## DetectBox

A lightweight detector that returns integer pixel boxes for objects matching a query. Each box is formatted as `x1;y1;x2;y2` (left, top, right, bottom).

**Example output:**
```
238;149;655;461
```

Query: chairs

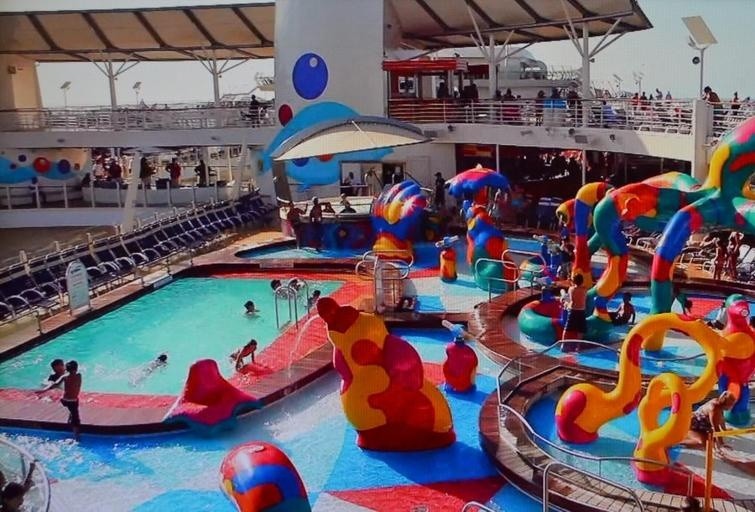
0;187;277;327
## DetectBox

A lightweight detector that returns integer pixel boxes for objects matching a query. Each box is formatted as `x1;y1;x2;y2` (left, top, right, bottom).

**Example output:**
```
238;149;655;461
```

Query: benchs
613;214;753;285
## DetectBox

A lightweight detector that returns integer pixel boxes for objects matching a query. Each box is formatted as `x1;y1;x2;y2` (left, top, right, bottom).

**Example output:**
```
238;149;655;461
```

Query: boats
375;42;587;98
131;74;279;189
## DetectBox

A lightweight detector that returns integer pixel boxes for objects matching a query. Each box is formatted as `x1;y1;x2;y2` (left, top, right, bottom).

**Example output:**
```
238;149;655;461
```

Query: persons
127;350;169;389
496;88;582;127
670;230;742;331
228;338;258;372
435;173;447;209
243;279;321;314
690;391;735;455
34;359;82;442
287;172;357;252
40;359;66;388
249;93;260;127
704;87;740;137
602;88;672;123
1;459;39;512
487;153;604;226
439;82;479;121
29;176;48;207
557;224;663;352
93;154;211;189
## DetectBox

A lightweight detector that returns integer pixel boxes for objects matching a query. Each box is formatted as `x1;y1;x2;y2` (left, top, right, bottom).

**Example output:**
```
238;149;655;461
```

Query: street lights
59;79;73;110
132;80;142;106
683;15;719;102
